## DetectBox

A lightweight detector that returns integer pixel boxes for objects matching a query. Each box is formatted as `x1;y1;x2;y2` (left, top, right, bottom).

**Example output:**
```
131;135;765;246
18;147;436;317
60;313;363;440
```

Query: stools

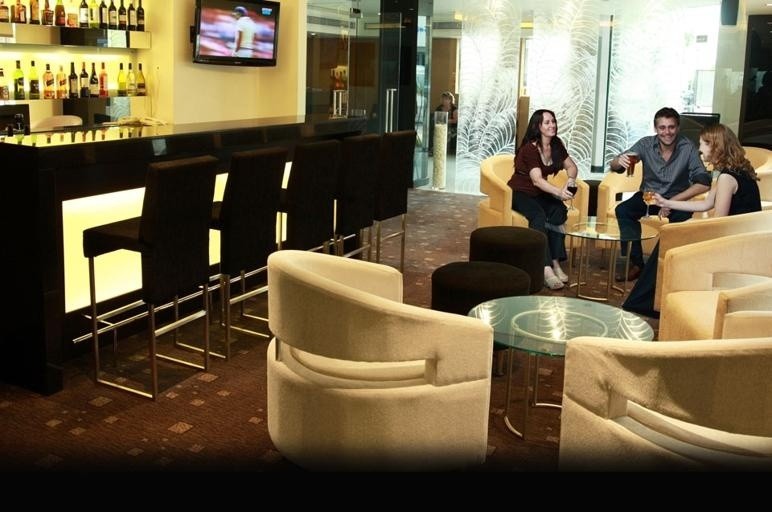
470;226;547;295
432;261;532;316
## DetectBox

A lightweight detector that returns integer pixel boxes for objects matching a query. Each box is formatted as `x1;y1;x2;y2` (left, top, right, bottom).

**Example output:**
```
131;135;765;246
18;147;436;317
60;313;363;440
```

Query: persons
622;124;763;320
429;92;458;156
609;107;712;283
233;4;259;59
506;108;579;291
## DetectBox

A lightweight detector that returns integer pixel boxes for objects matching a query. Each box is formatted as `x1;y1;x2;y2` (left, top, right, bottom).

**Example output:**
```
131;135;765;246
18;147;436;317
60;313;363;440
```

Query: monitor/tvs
192;0;280;67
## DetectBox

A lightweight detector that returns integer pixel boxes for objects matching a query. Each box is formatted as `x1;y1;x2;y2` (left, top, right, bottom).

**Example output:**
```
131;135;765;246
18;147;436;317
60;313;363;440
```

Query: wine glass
641;189;655;219
566;181;578;211
625;151;638;179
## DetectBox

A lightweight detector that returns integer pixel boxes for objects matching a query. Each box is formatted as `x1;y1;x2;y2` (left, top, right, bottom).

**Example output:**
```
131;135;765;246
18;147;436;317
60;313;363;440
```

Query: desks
0;114;373;396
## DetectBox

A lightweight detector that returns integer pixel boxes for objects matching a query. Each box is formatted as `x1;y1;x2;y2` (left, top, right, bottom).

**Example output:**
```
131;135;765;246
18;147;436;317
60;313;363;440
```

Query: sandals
544;274;565;289
553;267;569;282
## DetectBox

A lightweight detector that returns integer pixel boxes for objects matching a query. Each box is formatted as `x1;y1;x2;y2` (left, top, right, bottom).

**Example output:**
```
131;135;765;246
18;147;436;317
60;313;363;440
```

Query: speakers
720;1;739;25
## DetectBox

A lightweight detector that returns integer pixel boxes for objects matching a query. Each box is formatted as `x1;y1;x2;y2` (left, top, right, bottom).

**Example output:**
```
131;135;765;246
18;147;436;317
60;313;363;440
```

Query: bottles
0;0;148;101
14;114;26;133
0;127;148;146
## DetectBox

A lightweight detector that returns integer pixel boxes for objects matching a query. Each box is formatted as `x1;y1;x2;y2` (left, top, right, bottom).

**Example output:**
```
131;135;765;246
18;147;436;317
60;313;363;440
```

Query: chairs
267;249;494;471
479;154;590;266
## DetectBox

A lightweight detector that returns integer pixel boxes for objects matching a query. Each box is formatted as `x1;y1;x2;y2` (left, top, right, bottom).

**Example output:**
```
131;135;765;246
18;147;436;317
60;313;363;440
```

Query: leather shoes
615;264;641;282
